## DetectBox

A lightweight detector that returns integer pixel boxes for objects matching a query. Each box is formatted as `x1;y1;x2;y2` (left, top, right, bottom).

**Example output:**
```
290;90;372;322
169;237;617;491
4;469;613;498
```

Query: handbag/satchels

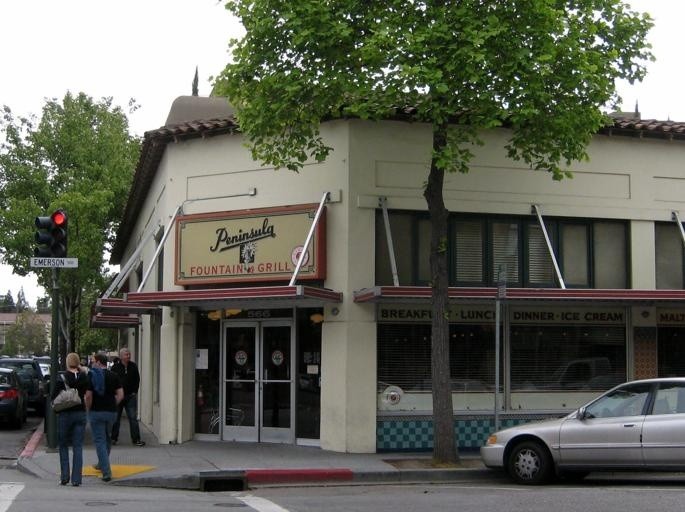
51;388;81;412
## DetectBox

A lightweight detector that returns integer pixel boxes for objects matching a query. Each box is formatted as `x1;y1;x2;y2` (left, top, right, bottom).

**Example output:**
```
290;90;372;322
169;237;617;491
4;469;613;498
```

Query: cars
0;354;65;430
483;376;685;484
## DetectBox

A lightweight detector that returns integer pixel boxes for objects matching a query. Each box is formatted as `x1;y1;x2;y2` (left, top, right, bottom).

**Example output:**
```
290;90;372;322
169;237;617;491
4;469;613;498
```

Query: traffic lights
50;211;69;257
34;215;51;256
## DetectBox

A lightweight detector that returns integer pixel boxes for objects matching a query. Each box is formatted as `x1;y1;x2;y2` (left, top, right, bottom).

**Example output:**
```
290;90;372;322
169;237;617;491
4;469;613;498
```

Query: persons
50;351;88;487
83;353;125;481
110;348;146;446
106;356;121;371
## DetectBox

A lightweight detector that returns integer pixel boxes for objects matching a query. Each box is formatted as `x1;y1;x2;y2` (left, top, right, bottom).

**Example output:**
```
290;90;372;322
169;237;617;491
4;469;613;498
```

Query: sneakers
61;479;80;486
134;440;146;446
93;463;111;481
